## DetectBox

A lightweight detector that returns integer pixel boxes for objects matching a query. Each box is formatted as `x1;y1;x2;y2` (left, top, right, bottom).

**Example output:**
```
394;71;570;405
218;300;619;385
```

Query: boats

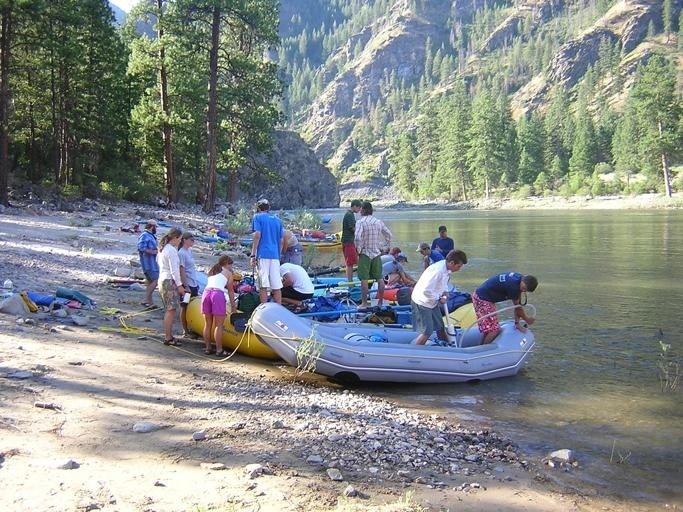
223;228;342;254
247;300;537;384
183;266;493;359
275;210;331;224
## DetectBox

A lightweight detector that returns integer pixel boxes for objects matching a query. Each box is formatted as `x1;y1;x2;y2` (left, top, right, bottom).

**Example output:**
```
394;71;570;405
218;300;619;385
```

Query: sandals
141;302;231;357
293;301;307;313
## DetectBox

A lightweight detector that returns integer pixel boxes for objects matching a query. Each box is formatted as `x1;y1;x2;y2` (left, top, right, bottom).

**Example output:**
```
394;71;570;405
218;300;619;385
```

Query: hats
147;219;159;226
398;253;408;262
416;243;430;252
182;232;193;238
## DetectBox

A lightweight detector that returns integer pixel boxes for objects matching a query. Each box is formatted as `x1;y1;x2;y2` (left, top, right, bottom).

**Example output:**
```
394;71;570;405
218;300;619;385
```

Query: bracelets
442;295;447;300
514;321;520;325
250;254;256;258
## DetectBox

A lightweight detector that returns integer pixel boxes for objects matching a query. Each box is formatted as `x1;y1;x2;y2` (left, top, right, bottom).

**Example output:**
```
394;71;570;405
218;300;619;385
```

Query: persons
354;202;394;308
158;227;187;347
381;254;417;288
248;198;286;308
469;270;539;344
414;240;446;268
198;254;239;358
136;218;159;309
408;249;468;345
429;225;456;259
176;232;200;340
277;259;315;312
340;199;363;282
281;226;304;266
378;247;402;264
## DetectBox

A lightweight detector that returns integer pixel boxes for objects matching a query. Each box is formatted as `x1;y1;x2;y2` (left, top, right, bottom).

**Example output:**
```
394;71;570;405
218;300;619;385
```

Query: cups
182;292;191;304
